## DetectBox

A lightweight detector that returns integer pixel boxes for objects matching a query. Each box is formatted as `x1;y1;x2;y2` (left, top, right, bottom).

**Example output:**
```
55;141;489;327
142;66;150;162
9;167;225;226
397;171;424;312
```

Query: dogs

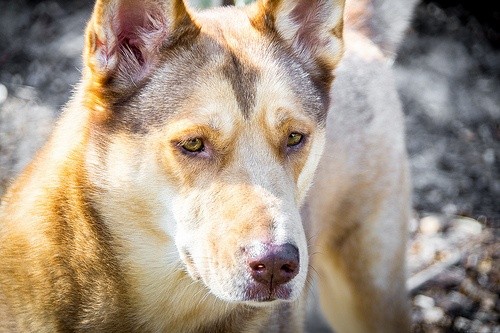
0;1;414;333
